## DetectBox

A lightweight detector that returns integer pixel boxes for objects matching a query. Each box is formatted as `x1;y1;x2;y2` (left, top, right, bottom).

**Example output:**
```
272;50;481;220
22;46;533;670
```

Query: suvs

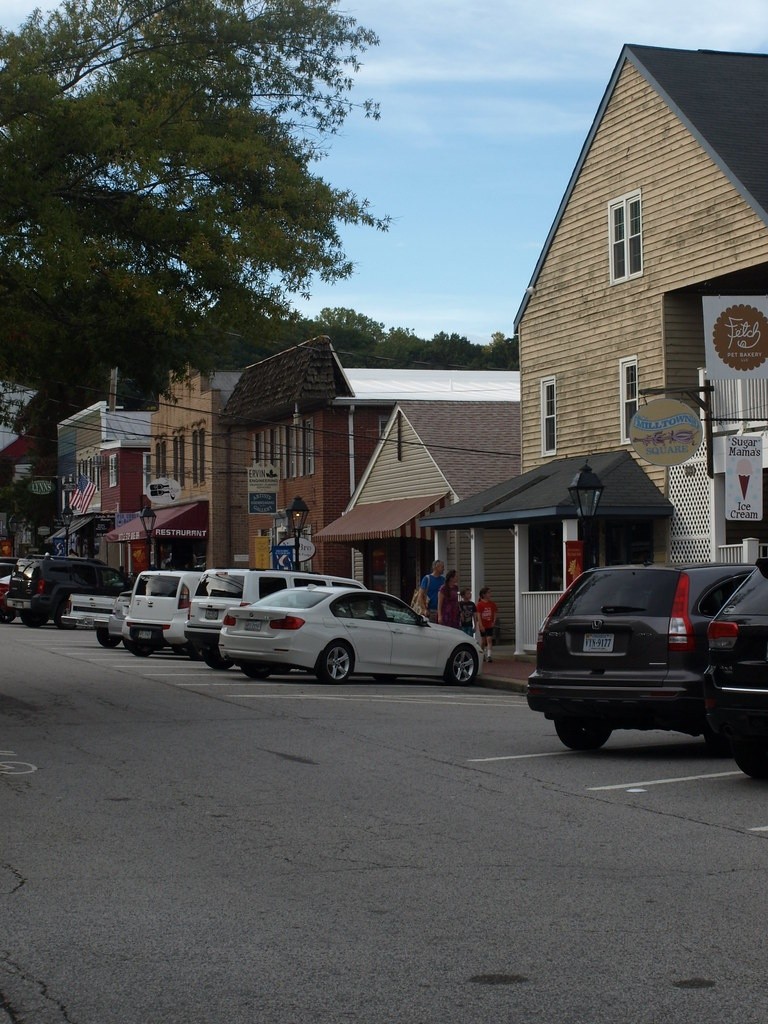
6;554;133;628
184;567;368;669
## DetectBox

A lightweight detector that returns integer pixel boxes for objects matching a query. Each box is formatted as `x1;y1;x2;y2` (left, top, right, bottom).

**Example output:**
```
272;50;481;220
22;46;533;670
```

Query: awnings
103;501;209;542
311;494;444;542
44;516;93;543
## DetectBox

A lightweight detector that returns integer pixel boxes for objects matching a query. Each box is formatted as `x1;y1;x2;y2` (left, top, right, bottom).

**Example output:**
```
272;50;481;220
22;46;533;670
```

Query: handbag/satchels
411;575;430;615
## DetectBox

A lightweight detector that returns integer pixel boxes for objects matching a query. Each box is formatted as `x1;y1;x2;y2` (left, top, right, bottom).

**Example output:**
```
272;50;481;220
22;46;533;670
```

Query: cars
525;559;757;750
108;590;132;634
217;584;486;686
121;571;206;662
0;557;19;623
702;559;768;779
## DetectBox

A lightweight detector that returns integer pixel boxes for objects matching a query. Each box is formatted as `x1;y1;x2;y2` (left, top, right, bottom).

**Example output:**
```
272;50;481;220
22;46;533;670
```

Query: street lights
61;506;74;557
566;466;606;572
286;495;309;571
139;506;158;570
8;514;19;556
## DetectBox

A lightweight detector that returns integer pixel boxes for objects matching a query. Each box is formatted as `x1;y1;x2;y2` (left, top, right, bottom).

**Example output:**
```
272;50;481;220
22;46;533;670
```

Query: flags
69;476;96;514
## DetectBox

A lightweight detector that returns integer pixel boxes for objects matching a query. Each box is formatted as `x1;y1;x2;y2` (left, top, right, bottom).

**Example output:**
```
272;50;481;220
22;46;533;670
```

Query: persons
438;569;459;628
458;587;477;638
475;587;497;662
119;566;125;577
67;549;79;557
419;560;445;624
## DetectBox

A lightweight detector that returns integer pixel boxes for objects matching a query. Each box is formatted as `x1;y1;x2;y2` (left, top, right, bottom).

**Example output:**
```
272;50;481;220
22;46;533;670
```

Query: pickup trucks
59;594;121;649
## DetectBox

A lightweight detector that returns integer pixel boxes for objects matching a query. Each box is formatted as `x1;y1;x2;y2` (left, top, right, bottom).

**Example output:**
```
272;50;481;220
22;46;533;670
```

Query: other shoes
484;655;492;662
